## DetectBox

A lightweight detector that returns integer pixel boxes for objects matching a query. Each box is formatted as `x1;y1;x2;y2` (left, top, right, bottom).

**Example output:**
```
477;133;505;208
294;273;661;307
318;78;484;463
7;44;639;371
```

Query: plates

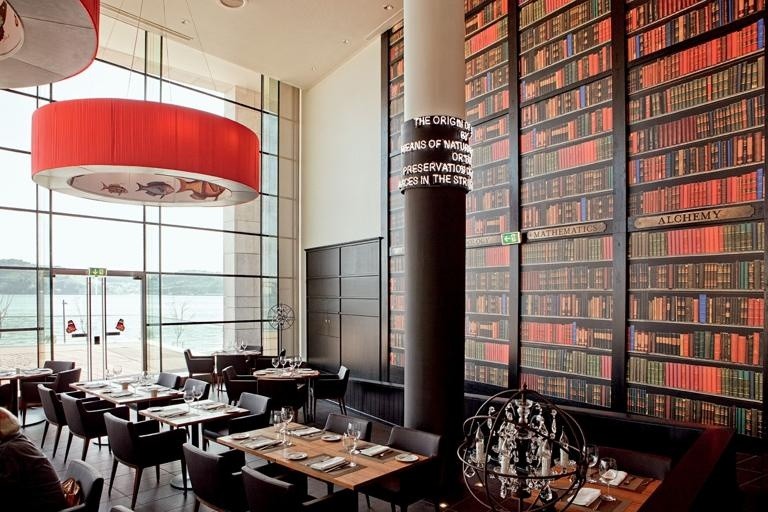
321;435;342;441
287;451;307;460
231;433;250;440
395;453;419;463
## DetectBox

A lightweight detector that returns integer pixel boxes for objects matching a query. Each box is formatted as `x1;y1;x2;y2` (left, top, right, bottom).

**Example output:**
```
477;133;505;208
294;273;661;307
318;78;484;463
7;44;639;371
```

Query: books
625;1;766;216
465;0;509;390
519;0;612;407
389;25;403;384
626;222;765;437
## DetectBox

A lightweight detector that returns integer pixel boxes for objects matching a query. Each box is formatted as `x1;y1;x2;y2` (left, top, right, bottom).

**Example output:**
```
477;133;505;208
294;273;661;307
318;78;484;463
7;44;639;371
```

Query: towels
567;487;601;507
599;469;629;487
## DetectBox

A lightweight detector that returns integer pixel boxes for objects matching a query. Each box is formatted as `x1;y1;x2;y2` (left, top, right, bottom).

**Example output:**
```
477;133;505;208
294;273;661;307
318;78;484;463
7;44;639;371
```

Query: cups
151;389;157;398
16;367;20;374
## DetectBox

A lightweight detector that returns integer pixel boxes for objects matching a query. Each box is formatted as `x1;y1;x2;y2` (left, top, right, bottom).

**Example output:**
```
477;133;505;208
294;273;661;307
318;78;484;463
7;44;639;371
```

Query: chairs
184;345;351;425
598;444;674;480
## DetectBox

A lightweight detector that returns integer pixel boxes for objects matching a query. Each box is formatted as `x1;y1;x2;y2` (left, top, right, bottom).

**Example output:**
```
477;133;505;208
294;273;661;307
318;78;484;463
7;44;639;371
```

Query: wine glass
582;444;599;482
233;337;247;353
103;365;156;392
599;457;618;502
183;384;203;416
342;432;357;467
272;353;303;376
347;421;361;455
271;405;294;449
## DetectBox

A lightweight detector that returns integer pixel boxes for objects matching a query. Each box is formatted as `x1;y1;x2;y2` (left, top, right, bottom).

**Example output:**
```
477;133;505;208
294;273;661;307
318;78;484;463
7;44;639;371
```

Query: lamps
0;0;101;90
32;0;261;207
457;382;587;511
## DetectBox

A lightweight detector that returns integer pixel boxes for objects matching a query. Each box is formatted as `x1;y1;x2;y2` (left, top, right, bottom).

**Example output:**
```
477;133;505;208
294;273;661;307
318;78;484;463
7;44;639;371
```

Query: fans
268;303;295;352
